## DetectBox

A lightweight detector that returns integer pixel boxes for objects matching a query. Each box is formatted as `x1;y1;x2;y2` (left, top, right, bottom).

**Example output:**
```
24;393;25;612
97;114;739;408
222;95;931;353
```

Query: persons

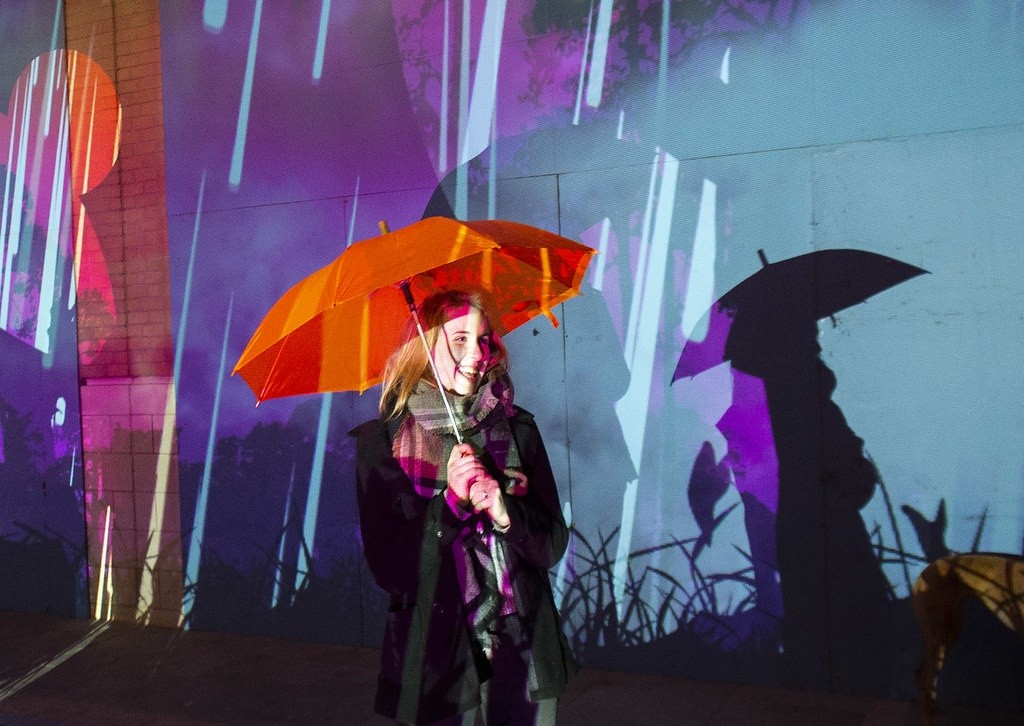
345;285;570;726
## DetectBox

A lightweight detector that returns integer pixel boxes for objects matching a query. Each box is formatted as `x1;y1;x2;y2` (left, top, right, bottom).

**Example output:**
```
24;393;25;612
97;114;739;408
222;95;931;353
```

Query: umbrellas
228;214;598;481
